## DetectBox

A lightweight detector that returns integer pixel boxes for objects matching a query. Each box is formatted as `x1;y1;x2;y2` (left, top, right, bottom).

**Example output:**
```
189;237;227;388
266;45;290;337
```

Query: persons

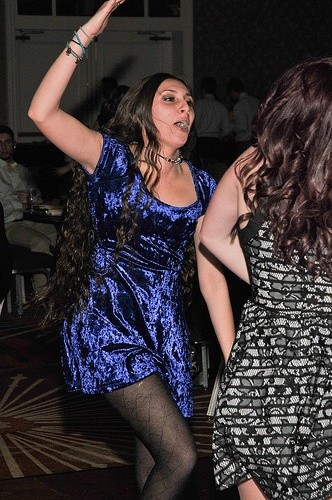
199;58;332;500
36;0;238;500
0;75;264;319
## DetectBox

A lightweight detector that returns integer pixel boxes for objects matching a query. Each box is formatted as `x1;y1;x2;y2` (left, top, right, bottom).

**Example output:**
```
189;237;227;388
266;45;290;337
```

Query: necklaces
148;146;184;166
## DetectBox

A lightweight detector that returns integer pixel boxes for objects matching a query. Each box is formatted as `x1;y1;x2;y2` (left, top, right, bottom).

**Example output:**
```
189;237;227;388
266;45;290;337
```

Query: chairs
0;201;52;318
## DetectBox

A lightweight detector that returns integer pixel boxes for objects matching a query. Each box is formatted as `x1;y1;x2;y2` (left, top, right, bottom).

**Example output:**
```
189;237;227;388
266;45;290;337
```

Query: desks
23;205;64;231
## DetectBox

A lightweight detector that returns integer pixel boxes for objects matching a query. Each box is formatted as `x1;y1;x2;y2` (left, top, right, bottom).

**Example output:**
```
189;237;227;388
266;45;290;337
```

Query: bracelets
65;25;95;64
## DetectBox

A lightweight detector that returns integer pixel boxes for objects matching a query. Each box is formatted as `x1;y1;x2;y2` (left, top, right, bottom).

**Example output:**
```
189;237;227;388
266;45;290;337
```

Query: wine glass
27;186;37;212
